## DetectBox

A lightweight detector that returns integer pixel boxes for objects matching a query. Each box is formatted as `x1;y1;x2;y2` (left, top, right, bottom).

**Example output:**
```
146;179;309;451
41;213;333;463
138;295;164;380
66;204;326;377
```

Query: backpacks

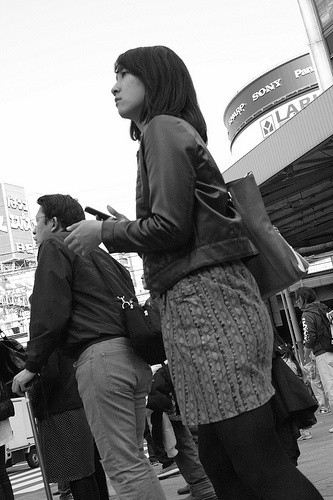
312;302;333;352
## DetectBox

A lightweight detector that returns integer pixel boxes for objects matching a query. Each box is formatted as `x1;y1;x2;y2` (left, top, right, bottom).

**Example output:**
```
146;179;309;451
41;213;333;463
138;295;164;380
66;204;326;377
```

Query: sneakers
157;462;180;478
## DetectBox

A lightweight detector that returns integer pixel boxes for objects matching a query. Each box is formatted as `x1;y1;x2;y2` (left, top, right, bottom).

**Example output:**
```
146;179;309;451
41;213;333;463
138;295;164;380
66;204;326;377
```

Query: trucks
3;396;40;469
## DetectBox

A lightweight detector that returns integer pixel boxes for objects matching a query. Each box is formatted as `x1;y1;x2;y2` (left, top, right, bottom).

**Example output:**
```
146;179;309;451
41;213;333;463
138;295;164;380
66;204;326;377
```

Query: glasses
30;216;44;228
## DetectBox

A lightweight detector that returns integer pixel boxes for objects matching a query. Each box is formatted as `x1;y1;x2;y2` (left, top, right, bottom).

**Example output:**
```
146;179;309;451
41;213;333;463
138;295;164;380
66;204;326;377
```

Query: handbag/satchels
0;338;35;399
121;300;167;365
225;171;309;301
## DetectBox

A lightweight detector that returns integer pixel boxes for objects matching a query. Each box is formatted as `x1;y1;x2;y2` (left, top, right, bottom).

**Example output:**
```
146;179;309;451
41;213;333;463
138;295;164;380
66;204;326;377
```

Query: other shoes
297;432;312;440
60;493;74;500
177;484;192;495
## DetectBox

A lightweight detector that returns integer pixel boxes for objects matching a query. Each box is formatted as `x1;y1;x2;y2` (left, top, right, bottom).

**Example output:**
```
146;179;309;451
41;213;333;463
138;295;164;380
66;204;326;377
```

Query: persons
62;46;323;500
272;328;312;440
0;328;26;500
144;360;216;500
295;286;333;434
12;195;165;500
